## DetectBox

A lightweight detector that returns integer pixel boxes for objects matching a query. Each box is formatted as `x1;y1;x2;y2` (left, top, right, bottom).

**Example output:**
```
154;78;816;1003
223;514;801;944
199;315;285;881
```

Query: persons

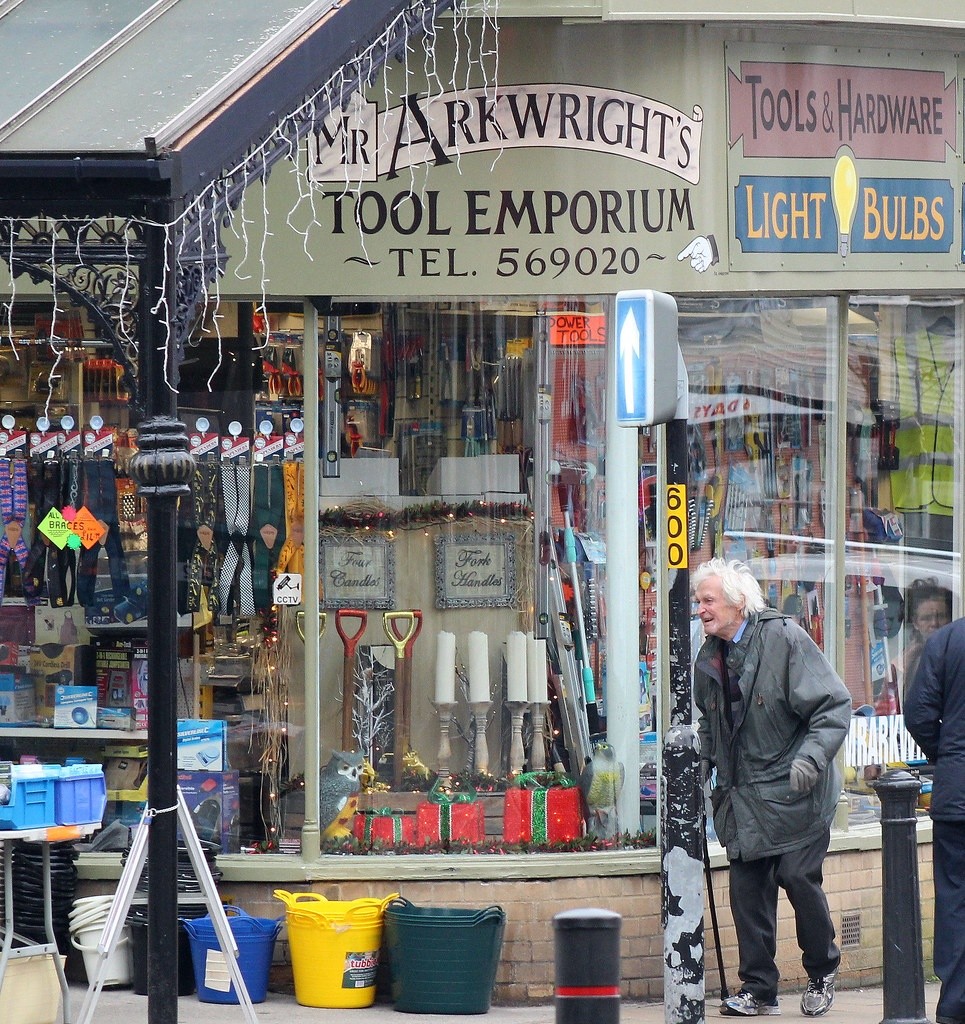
875;577;953;717
690;557;853;1017
904;615;965;1024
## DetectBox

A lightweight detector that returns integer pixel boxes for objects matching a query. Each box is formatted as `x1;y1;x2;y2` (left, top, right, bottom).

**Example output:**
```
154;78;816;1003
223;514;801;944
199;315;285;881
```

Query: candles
508;632;526;701
436;630;457;701
469;629;491;701
528;631;548;703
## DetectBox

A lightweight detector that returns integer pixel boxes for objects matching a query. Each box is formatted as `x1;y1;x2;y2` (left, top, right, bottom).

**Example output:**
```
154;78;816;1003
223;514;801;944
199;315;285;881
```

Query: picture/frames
434;531;519;609
317;536;397;609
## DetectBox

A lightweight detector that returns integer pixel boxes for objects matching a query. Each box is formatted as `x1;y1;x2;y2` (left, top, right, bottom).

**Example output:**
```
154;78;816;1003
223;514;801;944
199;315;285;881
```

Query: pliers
285;349;302;397
352;348;377;395
351;422;364;461
265;346;283;396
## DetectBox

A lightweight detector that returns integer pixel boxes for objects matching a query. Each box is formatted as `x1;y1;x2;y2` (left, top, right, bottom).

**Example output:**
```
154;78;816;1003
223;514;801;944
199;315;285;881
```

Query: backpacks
862;507;904;545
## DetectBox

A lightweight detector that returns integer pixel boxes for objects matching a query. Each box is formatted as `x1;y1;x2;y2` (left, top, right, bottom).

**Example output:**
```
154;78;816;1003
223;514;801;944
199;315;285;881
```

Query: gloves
789;754;819;795
701;759;714;786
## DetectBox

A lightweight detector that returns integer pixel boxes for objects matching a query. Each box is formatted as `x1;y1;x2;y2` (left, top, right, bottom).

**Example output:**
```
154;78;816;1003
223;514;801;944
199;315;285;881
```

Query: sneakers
800;967;841;1017
718;989;782;1016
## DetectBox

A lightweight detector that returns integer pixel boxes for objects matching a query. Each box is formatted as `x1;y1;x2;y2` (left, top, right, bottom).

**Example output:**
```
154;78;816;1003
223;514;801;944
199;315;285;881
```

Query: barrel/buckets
381;895;507;1016
273;888;400;1008
67;894;133;988
119;837;224;996
178;904;286;1005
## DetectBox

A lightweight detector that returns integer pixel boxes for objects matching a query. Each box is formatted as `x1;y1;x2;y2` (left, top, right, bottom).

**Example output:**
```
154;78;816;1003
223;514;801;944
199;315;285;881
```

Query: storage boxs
176;719;228;771
0;576;148;730
55;771;109;826
416;801;484;848
426;454;520;496
502;784;587;846
320;458;399;496
354;810;413;850
176;769;242;854
0;761;57;831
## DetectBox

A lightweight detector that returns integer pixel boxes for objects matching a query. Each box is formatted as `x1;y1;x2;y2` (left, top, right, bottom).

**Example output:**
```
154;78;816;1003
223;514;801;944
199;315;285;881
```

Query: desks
0;823;102;1024
